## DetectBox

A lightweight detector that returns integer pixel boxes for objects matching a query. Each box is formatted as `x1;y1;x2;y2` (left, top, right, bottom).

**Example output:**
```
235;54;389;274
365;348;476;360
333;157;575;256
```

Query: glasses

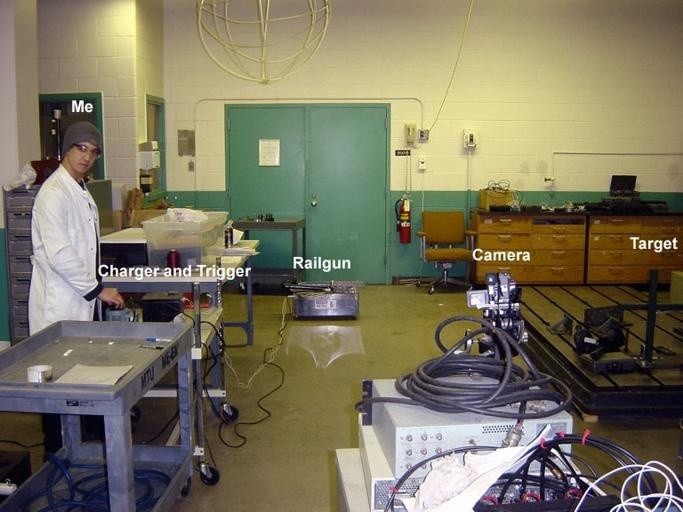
72;143;102;156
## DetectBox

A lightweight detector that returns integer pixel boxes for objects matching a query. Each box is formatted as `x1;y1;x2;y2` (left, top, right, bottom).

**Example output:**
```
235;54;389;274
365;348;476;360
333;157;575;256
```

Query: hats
62;121;101;158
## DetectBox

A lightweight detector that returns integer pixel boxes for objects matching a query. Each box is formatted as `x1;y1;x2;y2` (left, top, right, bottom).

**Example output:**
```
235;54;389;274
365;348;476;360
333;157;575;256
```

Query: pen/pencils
145;337;172;342
138;344;163;350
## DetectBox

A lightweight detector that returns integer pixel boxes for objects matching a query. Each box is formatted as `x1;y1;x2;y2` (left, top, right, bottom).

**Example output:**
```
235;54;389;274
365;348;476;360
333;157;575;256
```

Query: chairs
416;211;476;294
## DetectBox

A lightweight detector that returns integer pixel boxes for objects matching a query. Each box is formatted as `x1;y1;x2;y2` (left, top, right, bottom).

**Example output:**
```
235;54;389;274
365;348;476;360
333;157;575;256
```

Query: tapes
26;364;52;383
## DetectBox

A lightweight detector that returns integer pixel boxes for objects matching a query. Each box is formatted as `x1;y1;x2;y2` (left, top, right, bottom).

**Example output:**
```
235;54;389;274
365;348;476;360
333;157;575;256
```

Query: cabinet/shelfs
471;210;587;286
588;215;683;285
4;184;44;347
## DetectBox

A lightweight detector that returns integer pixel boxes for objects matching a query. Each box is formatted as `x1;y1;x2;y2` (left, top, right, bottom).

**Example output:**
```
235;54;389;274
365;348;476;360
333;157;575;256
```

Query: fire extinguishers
394;192;411;244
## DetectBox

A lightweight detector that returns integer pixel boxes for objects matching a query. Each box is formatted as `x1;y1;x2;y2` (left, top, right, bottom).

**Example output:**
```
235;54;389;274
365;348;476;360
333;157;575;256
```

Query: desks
102;239;260;417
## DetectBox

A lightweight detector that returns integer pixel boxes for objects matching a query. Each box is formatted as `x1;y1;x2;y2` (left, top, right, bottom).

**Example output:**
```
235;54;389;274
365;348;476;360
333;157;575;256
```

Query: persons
26;122;123;458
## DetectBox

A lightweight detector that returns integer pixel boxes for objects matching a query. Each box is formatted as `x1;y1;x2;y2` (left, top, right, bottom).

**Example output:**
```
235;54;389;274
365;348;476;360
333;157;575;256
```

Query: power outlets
418;130;429;140
